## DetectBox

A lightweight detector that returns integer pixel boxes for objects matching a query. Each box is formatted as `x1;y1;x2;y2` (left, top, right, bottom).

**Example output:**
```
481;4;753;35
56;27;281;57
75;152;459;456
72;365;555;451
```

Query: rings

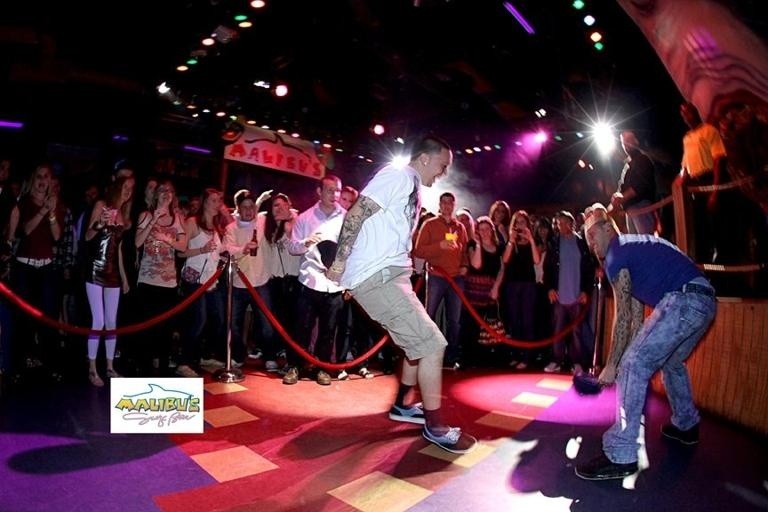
310;235;315;240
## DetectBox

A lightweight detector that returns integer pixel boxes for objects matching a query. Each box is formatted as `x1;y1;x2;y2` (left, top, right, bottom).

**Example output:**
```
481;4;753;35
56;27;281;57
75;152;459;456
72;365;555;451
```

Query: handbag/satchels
477;297;511;345
182;226;215;284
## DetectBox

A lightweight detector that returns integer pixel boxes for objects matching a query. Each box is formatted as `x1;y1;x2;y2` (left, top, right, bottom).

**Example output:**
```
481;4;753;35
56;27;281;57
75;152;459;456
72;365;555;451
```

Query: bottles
250;230;258;256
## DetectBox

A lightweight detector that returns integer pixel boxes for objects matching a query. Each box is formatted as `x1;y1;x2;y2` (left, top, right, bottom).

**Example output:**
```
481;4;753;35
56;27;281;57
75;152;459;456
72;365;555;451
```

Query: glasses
326;186;342;195
156;186;178;195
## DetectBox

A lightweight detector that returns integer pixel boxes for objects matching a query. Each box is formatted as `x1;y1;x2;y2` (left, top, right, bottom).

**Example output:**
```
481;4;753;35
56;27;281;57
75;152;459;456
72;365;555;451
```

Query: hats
573;362;605;396
194;186;220;218
316;239;338;271
229;188;255;216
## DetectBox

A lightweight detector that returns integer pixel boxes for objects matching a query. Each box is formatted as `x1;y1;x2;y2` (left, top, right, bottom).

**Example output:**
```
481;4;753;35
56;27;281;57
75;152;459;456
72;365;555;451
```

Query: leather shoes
316;367;336;386
282;364;301;384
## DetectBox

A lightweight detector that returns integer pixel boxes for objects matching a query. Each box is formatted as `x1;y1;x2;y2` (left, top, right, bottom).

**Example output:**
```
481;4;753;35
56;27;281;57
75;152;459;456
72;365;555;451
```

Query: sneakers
248;344;264;360
230;357;245;367
421;420;478;456
177;362;199;380
337;369;349;380
543;360;565;374
264;348;289;371
569;360;582;374
167;351;178;368
388;401;429;425
658;419;701;446
356;365;375;380
573;448;641;481
199;356;226;369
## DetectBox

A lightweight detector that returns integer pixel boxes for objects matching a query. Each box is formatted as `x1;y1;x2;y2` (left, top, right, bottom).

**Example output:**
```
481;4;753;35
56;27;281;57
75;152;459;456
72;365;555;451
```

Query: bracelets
91;221;104;233
148;221;155;228
48;216;57;226
475;243;482;248
508;239;516;247
38;211;46;218
199;247;203;254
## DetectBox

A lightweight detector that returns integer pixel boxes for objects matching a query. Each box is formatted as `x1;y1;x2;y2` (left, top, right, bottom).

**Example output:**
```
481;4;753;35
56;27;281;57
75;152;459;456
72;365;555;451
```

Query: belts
676;283;717;299
16;255;54;268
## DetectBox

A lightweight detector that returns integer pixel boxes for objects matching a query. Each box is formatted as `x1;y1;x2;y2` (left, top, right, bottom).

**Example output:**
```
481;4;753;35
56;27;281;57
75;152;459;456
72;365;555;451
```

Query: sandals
87;370;105;388
107;368;121;381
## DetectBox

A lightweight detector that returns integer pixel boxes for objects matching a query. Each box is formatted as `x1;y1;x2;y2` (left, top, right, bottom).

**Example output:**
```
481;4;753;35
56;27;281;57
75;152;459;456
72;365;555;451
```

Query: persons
4;161;68;383
188;198;202;214
84;175;131;388
718;99;768;301
490;199;512;257
408;212;436;310
74;182;100;260
283;174;347;385
677;100;727;284
253;189;300;387
454;209;478;244
49;177;79;349
606;130;661;235
134;180;188;377
575;213;718;480
224;189;300;374
178;187;242;378
533;216;556;286
367;299;397;374
491;211;559;371
324;133;478;455
464;216;528;372
335;186;375;382
112;159;141;360
413;192;470;371
543;211;597;377
140;179;159;213
